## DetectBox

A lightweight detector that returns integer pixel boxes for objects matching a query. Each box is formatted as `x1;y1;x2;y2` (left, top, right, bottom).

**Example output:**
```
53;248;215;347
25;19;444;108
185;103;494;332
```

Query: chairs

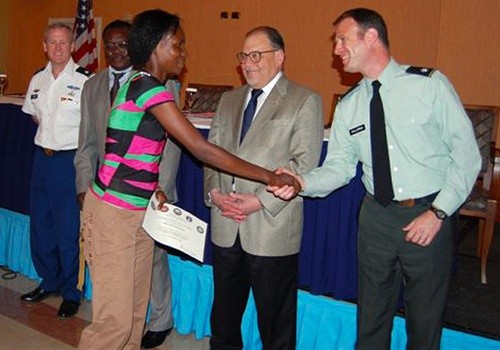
183;83;500;284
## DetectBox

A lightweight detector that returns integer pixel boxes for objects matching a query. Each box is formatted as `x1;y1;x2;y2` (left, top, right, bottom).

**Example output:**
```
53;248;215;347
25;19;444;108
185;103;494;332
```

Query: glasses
236;49;278;63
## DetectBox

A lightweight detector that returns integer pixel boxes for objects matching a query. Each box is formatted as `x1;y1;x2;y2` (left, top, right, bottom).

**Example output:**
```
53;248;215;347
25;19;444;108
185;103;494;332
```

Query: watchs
430;207;447;221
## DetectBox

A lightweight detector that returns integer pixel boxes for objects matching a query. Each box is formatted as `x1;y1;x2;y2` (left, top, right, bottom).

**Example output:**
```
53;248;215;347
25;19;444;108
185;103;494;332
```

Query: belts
389;191;440;206
40;147;63;156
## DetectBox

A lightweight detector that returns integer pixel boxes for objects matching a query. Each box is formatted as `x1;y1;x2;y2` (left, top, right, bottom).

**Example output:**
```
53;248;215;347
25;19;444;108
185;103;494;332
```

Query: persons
76;9;302;350
74;20;183;349
266;7;482;350
204;26;324;350
19;24;96;319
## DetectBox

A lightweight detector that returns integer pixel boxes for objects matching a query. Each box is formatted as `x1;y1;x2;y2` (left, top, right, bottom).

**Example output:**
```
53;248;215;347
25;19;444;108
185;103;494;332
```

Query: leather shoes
140;327;172;350
19;287;61;303
58;300;80;320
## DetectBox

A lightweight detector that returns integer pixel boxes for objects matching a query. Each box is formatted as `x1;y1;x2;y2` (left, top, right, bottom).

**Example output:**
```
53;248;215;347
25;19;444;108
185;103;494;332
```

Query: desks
0;93;500;350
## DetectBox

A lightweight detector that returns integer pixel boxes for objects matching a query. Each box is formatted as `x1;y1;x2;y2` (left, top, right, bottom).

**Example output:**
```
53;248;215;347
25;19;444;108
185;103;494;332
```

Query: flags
71;0;99;73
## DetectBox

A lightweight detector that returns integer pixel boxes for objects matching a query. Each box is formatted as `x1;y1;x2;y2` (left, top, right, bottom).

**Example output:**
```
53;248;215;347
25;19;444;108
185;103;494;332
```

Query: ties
230;88;263;185
369;80;396;207
110;72;127;107
